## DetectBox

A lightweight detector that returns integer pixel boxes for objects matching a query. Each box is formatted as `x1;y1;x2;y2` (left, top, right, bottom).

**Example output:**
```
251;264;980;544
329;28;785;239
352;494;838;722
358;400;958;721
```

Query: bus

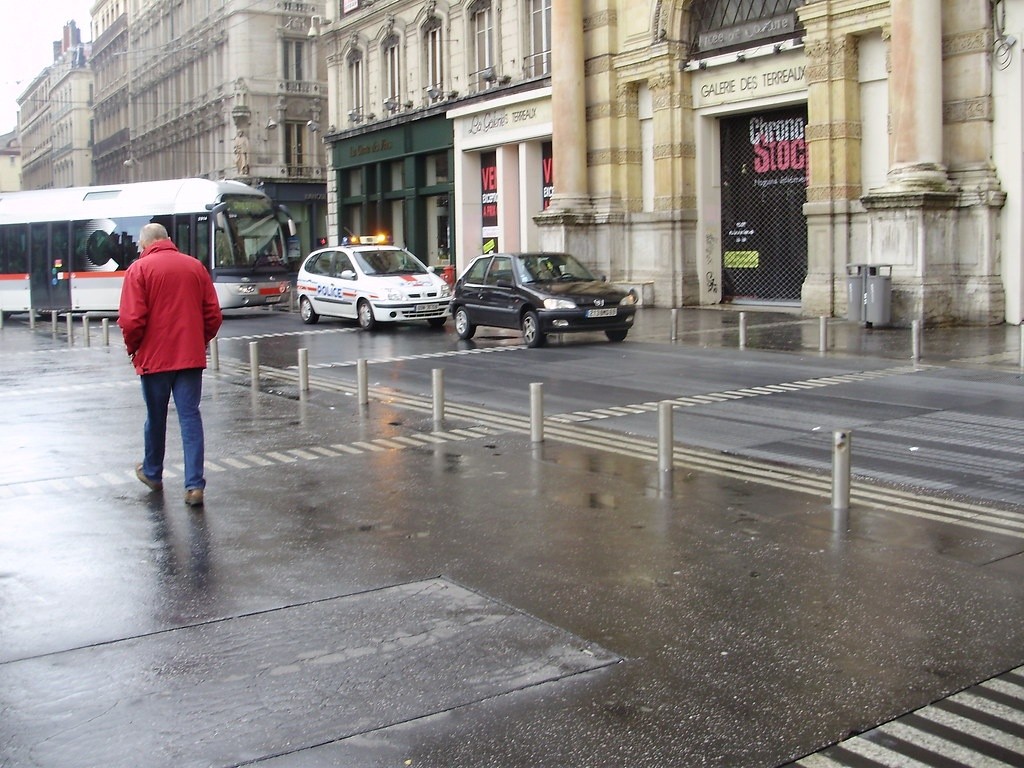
0;178;297;321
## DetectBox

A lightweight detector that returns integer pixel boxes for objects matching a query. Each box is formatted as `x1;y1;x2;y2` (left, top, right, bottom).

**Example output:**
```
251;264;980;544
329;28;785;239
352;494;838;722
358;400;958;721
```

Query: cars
296;237;451;330
448;252;636;347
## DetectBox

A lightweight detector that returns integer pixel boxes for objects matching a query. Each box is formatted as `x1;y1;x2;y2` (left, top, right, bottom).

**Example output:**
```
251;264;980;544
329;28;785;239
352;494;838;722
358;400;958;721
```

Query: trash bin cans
844;262;867;324
865;264;892;329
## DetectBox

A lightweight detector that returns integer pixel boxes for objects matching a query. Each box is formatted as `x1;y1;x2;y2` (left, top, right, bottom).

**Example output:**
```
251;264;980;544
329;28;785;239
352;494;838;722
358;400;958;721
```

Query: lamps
482;66;511;86
699;55;707;71
426;86;458;99
348;109;375;122
265;116;277;130
384;98;413;110
736;49;746;63
773;38;788;54
123;157;143;168
307;120;322;136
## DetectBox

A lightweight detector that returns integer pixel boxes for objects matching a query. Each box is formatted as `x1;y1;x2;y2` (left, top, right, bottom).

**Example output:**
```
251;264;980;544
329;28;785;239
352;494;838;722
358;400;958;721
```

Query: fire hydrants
442;265;455;289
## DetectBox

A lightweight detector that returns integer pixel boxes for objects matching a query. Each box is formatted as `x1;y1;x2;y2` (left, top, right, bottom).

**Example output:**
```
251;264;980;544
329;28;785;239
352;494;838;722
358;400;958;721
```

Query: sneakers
136;463;163;491
184;488;203;506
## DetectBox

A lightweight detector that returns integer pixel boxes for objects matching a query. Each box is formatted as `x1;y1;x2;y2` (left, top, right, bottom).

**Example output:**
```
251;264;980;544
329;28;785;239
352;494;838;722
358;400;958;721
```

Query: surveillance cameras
308;27;318;38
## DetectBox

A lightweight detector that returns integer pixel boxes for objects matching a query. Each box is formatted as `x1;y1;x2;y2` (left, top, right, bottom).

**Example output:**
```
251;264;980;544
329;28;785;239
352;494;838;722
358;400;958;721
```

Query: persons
117;223;222;507
0;237;86;306
234;243;247;265
537;259;557;281
